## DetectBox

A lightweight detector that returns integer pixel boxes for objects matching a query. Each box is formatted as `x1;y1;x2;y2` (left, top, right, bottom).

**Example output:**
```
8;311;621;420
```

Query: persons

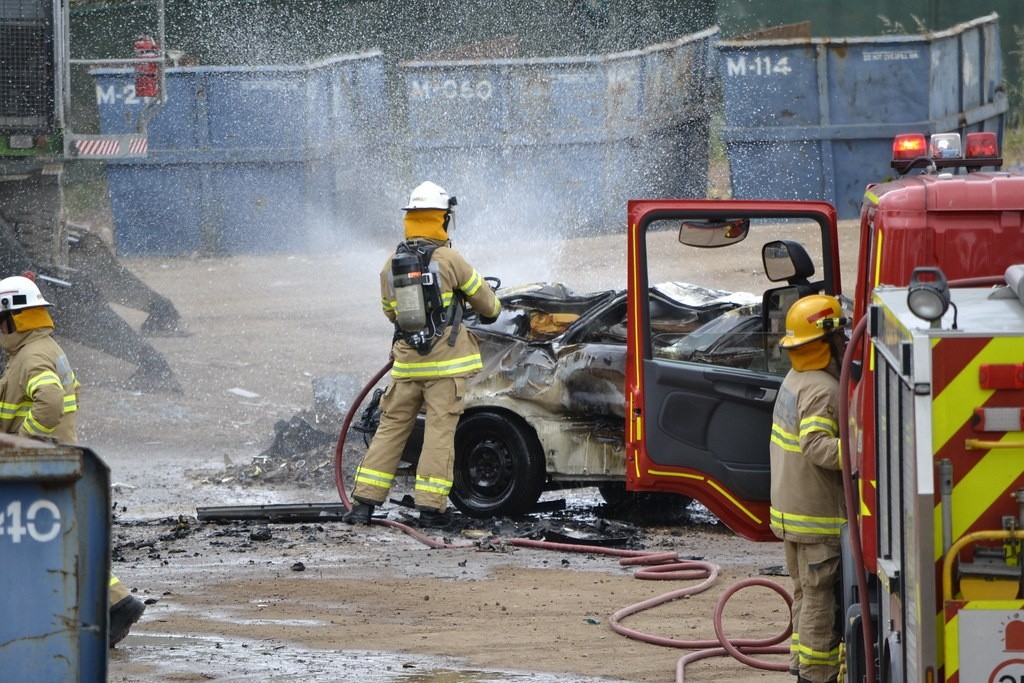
0;275;145;647
769;294;851;683
343;182;502;531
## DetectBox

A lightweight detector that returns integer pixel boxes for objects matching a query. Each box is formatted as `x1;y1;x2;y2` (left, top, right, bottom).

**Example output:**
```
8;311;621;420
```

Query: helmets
400;180;458;212
776;293;851;352
0;275;56;315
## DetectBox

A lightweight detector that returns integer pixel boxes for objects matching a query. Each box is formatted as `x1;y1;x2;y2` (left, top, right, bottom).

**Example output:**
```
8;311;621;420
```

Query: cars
351;281;857;521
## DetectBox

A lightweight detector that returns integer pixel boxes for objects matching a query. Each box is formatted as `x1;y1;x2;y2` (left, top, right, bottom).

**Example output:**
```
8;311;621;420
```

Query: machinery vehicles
0;0;194;399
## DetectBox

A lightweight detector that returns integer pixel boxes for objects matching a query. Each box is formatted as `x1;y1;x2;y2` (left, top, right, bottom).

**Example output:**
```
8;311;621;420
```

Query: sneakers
417;508;456;531
342;499;375;527
109;594;148;649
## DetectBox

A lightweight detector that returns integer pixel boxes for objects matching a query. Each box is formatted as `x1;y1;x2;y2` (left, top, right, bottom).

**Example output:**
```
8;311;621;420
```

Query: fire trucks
626;133;1024;683
